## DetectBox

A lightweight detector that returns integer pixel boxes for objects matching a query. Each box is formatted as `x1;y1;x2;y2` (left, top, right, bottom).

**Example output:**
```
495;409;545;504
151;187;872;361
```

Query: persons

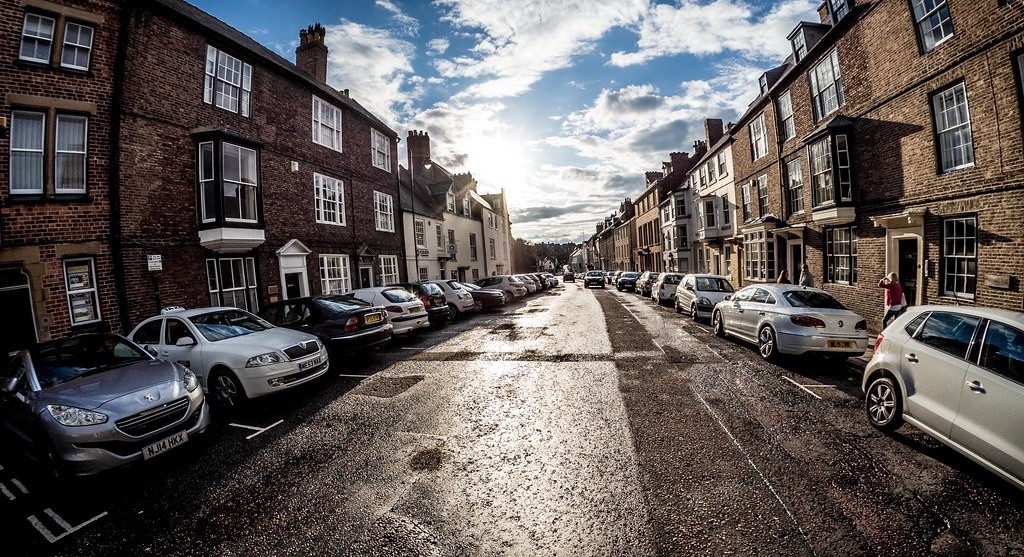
878;273;902;329
777;270;791;284
800;265;814;287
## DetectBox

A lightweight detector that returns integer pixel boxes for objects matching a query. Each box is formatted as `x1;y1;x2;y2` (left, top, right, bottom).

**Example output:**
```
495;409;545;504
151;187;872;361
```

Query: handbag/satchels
900;292;907;306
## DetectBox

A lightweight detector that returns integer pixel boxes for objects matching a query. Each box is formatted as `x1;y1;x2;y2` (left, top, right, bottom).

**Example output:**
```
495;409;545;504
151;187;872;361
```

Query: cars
1;330;212;487
525;273;543;290
470;274;528;305
460;281;509;304
516;273;537;297
384;280;450;325
461;285;506;315
615;272;644;293
420;278;475;322
674;273;739;323
603;271;615;285
536;272;551;291
651;271;688;305
562;272;576;283
113;306;331;418
711;281;869;363
634;270;662;296
543;271;558;287
228;295;394;362
862;304;1024;489
336;286;431;341
611;271;622;286
556;265;586;279
583;270;607;289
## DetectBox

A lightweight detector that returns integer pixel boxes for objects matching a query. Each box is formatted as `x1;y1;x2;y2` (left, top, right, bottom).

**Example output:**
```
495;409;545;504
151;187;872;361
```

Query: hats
801;264;808;269
